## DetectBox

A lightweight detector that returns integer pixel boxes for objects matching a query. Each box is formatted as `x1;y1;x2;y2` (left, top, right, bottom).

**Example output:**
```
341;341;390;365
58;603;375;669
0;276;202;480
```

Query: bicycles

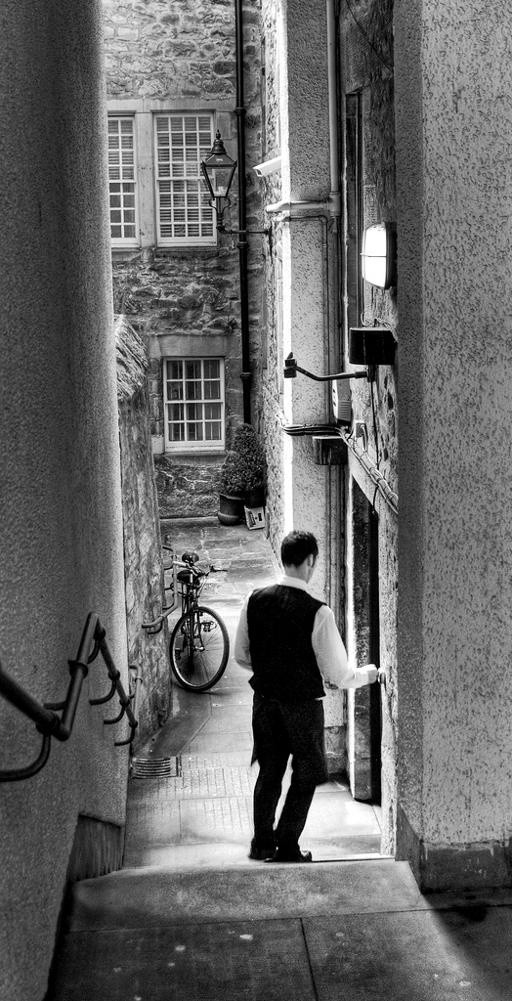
170;552;232;694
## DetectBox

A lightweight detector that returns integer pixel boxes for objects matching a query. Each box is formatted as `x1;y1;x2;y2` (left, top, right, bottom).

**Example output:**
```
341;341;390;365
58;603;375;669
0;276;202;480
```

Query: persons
231;529;378;863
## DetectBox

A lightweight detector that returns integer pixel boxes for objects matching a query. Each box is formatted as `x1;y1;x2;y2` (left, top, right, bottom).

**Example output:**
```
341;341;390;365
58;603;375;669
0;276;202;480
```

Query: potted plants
217;423;267;529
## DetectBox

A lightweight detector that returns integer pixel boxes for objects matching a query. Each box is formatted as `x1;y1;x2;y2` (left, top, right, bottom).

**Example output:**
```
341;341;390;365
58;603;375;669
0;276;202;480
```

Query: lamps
282;326;389;383
361;219;392;290
202;132;271;237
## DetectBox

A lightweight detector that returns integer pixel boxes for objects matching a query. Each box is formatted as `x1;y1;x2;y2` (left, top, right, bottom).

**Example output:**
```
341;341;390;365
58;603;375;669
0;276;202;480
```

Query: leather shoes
274;850;312;862
249;847;275;860
